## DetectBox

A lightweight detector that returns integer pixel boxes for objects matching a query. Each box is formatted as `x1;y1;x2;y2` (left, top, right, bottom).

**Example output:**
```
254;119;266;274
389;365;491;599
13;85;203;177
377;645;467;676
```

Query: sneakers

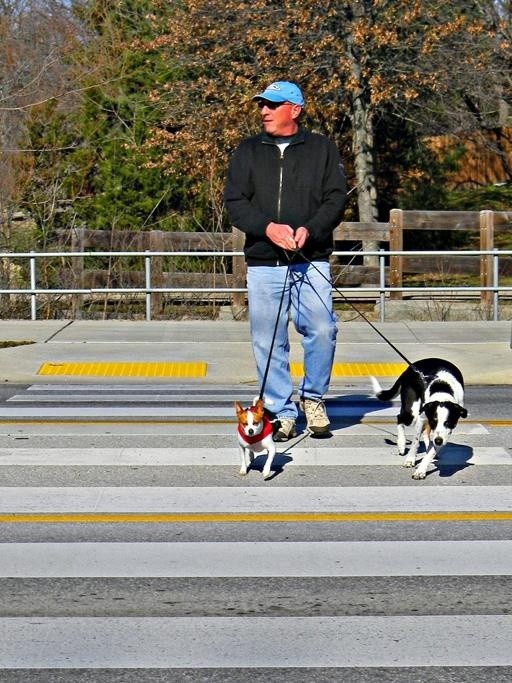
272;418;299;442
299;397;331;436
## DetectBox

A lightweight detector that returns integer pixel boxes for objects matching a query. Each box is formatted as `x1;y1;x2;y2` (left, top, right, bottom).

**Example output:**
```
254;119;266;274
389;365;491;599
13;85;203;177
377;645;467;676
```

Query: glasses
258;101;292;109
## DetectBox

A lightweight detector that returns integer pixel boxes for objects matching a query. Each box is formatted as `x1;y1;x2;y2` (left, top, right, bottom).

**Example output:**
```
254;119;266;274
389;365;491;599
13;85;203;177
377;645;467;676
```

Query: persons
221;81;349;440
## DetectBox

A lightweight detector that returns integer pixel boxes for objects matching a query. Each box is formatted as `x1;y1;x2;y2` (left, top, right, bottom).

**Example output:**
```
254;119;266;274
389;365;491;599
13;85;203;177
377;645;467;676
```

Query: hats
252;81;304;105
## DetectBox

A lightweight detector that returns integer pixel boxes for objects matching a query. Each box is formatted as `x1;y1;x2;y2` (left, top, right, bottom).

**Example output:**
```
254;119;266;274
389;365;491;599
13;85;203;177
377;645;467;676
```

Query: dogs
371;357;468;480
235;399;282;477
